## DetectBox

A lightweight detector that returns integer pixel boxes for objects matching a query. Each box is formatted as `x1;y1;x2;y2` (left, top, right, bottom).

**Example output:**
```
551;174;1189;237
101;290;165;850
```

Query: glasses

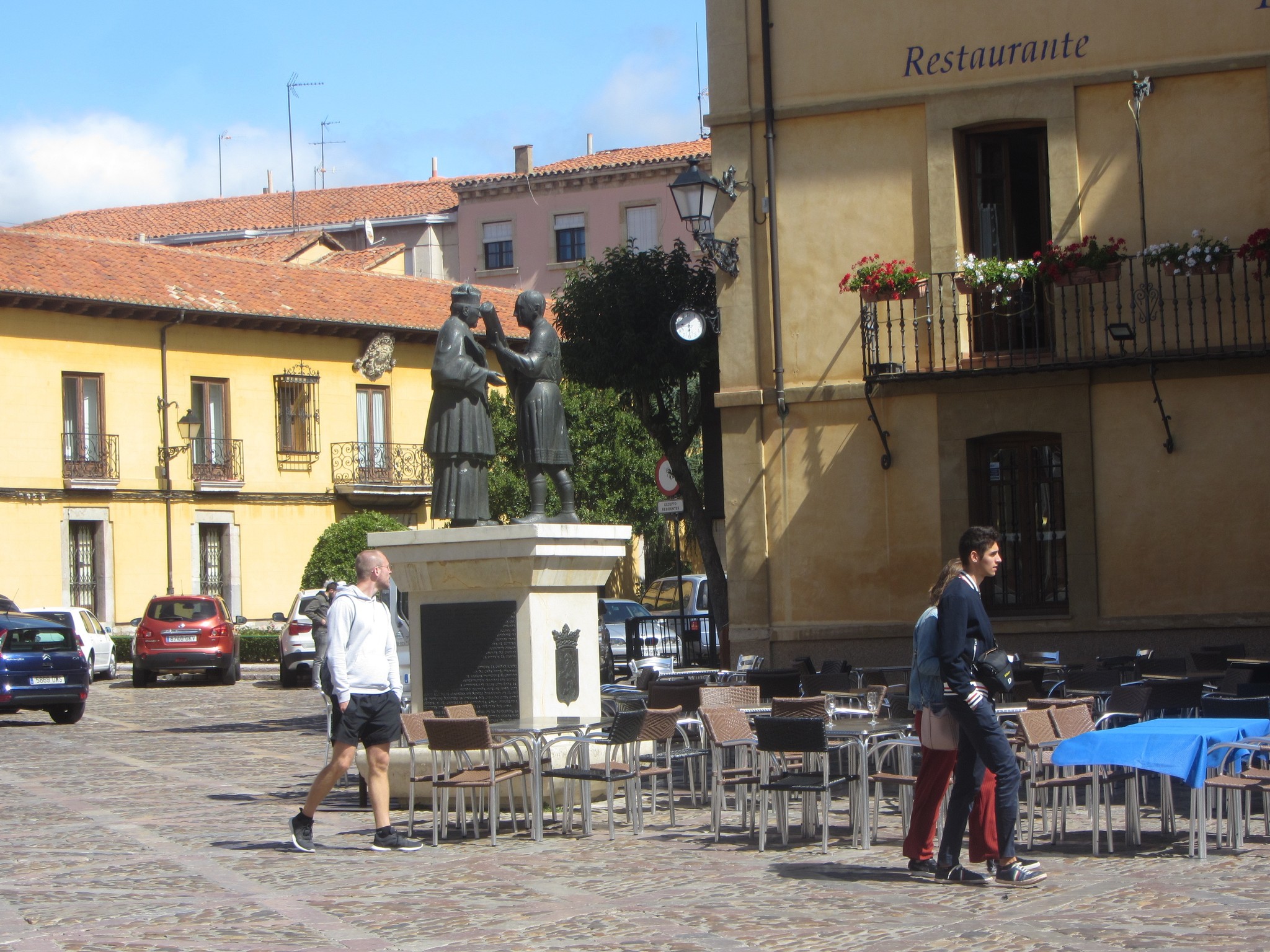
372;563;393;572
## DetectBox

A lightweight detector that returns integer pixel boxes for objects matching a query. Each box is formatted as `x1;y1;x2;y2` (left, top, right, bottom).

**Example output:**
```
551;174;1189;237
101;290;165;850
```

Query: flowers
1235;227;1270;281
1033;234;1129;283
838;254;931;301
955;250;1042;309
1136;227;1232;279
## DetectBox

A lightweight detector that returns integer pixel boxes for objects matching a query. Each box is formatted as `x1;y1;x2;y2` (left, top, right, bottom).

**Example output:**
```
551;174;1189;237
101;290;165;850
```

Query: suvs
130;594;247;688
639;574;727;669
273;587;343;688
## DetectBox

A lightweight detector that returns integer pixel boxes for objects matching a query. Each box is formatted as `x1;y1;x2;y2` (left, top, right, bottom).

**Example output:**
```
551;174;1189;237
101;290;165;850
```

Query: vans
381;573;411;703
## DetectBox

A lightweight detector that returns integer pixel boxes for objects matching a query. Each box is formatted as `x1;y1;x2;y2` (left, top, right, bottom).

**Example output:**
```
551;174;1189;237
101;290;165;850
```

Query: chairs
611;610;623;621
321;690;349;787
398;648;1270;859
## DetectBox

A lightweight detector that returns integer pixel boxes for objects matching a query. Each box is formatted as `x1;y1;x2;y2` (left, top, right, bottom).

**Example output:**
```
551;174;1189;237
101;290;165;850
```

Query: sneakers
934;864;993;887
289;809;316;852
995;862;1047;885
372;827;423;851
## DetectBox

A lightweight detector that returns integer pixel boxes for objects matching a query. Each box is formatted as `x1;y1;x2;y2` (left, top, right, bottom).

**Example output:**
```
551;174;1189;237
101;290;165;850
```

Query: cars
0;595;89;725
598;598;683;674
21;607;116;685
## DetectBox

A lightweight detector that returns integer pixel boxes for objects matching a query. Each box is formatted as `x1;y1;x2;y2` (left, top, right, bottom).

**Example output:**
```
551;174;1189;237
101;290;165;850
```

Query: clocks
668;302;721;346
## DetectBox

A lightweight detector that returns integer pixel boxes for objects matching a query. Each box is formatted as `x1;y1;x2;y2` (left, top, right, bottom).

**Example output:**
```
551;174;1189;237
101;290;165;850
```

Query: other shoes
987;852;1040;873
911;857;940;878
315;684;321;690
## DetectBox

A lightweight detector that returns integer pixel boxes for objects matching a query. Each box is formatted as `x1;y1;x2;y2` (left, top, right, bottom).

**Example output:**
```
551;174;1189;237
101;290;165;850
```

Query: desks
850;665;912;688
1064;682;1113;716
732;702;772;811
501;716;633;833
1051;726;1250;859
489;716;588;842
824;724;907;850
632;667;720;684
820;688;866;719
1125;718;1270;838
1024;662;1084;700
601;691;648;764
398;691;411;748
992;701;1029;727
825;718;916;830
1139;672;1227;687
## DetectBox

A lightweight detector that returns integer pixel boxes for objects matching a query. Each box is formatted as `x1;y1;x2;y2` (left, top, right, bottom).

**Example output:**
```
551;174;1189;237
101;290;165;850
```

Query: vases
1054;259;1122;287
858;278;929;303
1163;252;1234;276
953;274;1024;295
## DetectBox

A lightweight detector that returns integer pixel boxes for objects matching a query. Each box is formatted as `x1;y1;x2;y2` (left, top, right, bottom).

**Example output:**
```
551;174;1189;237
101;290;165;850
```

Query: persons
423;284;507;528
488;291;581;523
935;526;1049;884
287;548;425;853
304;581;339;692
906;556;1041;878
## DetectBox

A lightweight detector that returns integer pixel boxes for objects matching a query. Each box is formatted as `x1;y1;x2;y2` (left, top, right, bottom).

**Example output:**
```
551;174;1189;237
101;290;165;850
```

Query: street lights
163;409;203;596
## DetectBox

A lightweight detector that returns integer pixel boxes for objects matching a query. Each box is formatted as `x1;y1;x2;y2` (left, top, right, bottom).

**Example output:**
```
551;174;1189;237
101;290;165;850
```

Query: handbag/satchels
921;707;959;751
977;647;1015;694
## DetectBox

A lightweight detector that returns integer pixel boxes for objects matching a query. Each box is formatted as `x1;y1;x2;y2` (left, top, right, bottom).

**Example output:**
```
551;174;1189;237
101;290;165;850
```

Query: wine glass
824;694;836;726
867;692;880;724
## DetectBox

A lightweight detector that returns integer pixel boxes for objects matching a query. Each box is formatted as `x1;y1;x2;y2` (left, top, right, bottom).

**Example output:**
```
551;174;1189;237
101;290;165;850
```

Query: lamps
667;155;741;279
158;409;203;467
1108;322;1134;341
